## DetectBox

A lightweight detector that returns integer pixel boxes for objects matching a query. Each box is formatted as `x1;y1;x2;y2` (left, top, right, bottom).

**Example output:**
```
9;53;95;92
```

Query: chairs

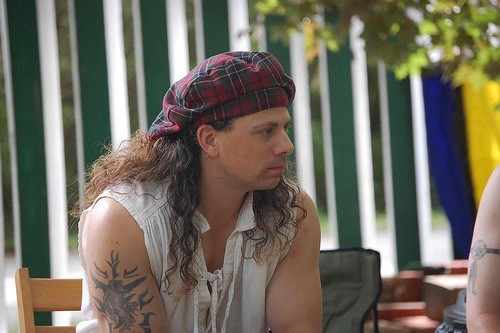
15;268;82;333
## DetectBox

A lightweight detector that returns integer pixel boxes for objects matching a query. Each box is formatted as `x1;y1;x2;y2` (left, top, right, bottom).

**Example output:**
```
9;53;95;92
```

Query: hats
146;50;296;140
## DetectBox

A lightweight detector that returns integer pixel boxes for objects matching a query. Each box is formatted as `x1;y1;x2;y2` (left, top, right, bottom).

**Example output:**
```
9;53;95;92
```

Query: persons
433;166;500;333
67;50;323;333
